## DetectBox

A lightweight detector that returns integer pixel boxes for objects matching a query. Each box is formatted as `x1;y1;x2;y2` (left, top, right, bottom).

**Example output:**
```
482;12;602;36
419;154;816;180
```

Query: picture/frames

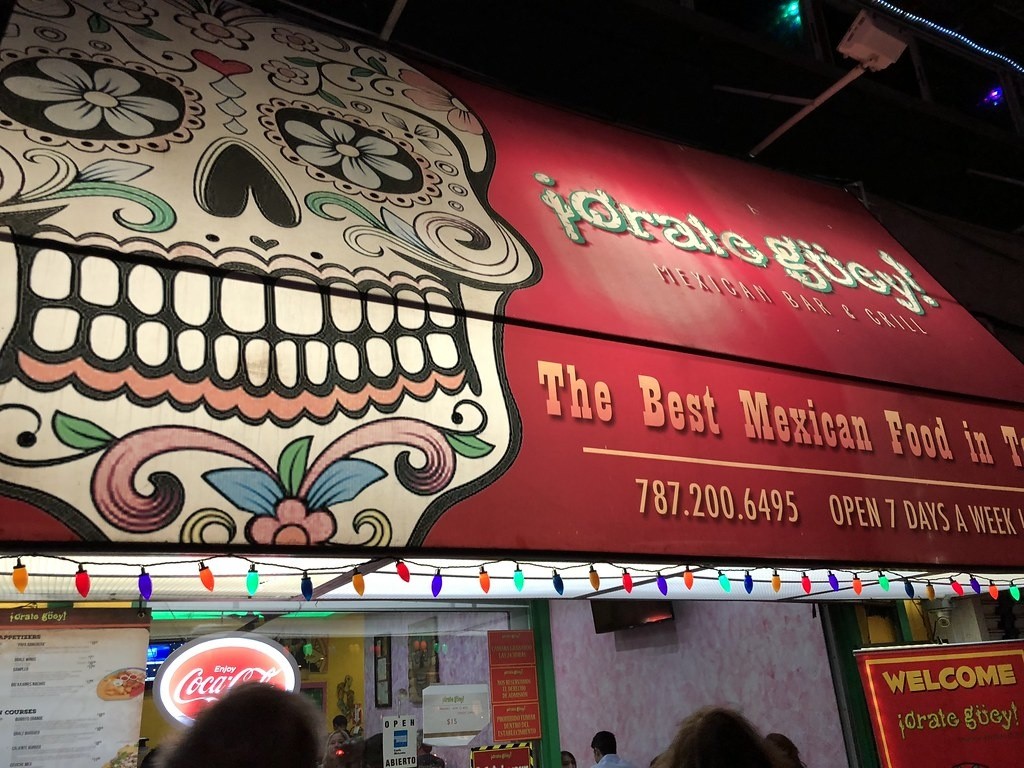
374;633;392;708
299;680;326;714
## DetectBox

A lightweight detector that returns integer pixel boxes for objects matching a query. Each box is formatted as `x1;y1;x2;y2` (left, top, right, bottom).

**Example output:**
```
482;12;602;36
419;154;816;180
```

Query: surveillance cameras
936;609;951;627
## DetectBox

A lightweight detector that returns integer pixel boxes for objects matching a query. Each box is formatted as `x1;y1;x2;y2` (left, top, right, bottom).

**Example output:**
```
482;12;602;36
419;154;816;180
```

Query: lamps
742;10;908;157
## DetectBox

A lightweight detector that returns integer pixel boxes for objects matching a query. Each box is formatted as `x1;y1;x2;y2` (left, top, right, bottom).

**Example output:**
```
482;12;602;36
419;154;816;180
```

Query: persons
417;729;445;768
561;751;577;768
765;733;802;768
168;680;320;768
590;731;637;768
332;716;352;737
320;729;351;768
656;708;791;768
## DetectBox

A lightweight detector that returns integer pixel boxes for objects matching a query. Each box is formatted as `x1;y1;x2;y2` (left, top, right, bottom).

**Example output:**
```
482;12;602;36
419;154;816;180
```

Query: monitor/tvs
144;638;195;690
590;599;675;634
